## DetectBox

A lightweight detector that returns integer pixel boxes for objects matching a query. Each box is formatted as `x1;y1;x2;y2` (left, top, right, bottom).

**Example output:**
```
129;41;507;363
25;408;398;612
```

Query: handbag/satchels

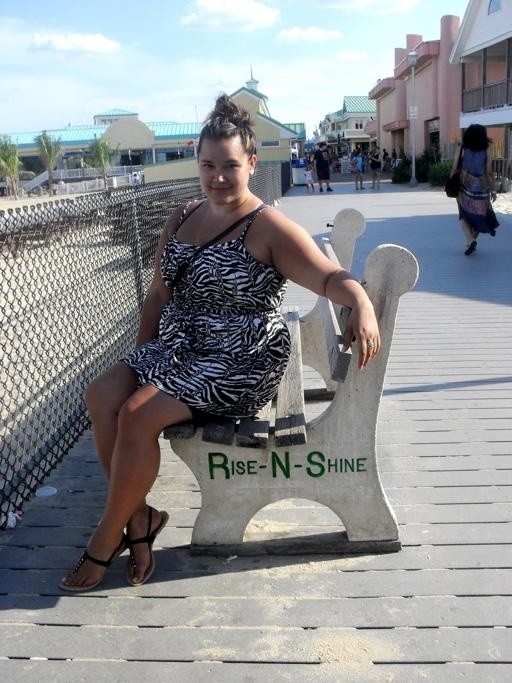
444;167;462;197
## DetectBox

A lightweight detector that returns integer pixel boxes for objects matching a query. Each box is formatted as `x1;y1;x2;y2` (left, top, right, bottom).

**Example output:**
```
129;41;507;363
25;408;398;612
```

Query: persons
62;91;383;598
303;141;404;193
448;123;499;255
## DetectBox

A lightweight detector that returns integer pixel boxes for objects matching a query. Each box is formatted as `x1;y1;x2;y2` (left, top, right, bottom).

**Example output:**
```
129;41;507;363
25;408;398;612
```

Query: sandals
129;505;169;586
57;532;127;593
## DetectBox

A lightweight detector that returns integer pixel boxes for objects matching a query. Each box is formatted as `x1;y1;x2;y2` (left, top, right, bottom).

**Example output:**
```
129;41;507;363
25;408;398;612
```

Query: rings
365;338;376;351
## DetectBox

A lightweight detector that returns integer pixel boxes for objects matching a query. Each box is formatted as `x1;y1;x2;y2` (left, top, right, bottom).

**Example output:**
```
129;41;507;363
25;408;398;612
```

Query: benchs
159;206;423;560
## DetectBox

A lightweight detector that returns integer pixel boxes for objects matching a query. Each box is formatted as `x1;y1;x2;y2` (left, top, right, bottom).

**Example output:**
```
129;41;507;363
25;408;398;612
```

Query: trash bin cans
291;158;307;186
28;190;34;197
53;188;58;195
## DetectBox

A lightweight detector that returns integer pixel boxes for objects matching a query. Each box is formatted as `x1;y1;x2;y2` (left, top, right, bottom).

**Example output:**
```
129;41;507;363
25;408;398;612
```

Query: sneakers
307;187;380;193
464;239;477;256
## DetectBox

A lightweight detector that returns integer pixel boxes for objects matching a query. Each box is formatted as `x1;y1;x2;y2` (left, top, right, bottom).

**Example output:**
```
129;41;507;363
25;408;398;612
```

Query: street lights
407;49;420;186
